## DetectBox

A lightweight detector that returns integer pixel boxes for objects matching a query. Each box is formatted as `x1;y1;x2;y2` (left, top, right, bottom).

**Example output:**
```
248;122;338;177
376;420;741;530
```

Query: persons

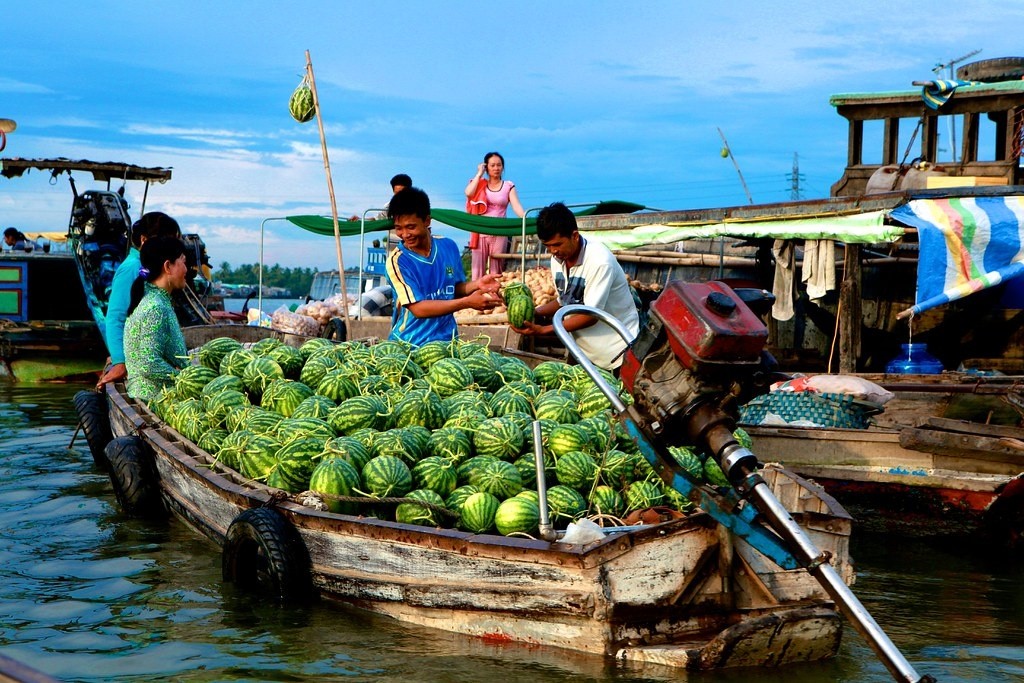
3;227;43;251
385;187;506;352
506;200;641;381
464;150;527;282
97;211;181;390
123;235;192;413
347;174;413;221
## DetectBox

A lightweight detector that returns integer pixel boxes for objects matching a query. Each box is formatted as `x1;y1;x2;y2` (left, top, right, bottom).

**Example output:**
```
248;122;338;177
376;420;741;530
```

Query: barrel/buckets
885;341;946;376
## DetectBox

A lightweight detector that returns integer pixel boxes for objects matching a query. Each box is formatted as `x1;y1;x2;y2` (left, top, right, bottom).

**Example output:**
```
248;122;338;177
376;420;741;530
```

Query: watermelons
142;283;755;533
289;87;315;122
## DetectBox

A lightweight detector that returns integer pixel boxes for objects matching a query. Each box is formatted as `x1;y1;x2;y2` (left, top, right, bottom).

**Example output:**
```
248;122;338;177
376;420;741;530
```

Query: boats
97;321;855;671
0;154;172;388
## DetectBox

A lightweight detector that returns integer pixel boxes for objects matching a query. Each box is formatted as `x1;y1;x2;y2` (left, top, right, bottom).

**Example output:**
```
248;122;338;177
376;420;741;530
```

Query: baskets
735;389;884;430
